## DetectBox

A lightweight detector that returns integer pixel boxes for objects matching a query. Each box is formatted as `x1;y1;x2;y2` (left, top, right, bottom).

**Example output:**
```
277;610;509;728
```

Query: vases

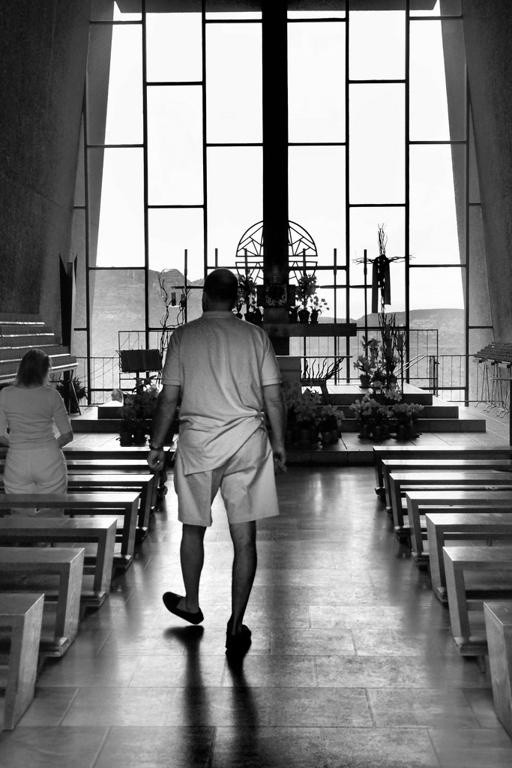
115;384;160;420
279;299;424;427
360;421;415;442
285;427;339;451
234;273;258;311
293;275;330;313
236;311;255;322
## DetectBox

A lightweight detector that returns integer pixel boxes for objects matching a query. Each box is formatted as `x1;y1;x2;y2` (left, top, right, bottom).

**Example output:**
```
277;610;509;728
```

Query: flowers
234;273;258;311
293;275;330;313
115;384;160;420
279;299;424;427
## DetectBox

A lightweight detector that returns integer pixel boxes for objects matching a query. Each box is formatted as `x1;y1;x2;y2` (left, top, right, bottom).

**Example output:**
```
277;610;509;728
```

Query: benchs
373;437;509;539
405;492;511;560
0;446;169;544
1;517;117;609
0;594;44;726
0;547;86;658
484;603;511;733
425;513;511;605
1;492;140;570
444;546;511;654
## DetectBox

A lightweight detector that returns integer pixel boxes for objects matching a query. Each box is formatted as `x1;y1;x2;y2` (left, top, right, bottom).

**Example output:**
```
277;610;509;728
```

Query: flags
360;421;415;442
285;427;339;451
236;311;255;322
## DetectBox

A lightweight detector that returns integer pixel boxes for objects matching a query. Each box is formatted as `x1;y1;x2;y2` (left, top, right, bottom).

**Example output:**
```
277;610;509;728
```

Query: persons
148;268;288;650
0;348;74;578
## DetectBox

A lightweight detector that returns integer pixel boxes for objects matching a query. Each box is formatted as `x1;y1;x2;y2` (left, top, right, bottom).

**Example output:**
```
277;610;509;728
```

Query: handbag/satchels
148;444;164;450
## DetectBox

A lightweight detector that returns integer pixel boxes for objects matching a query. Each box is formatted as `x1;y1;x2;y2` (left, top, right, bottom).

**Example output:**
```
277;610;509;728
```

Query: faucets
162;591;205;625
225;624;252;649
